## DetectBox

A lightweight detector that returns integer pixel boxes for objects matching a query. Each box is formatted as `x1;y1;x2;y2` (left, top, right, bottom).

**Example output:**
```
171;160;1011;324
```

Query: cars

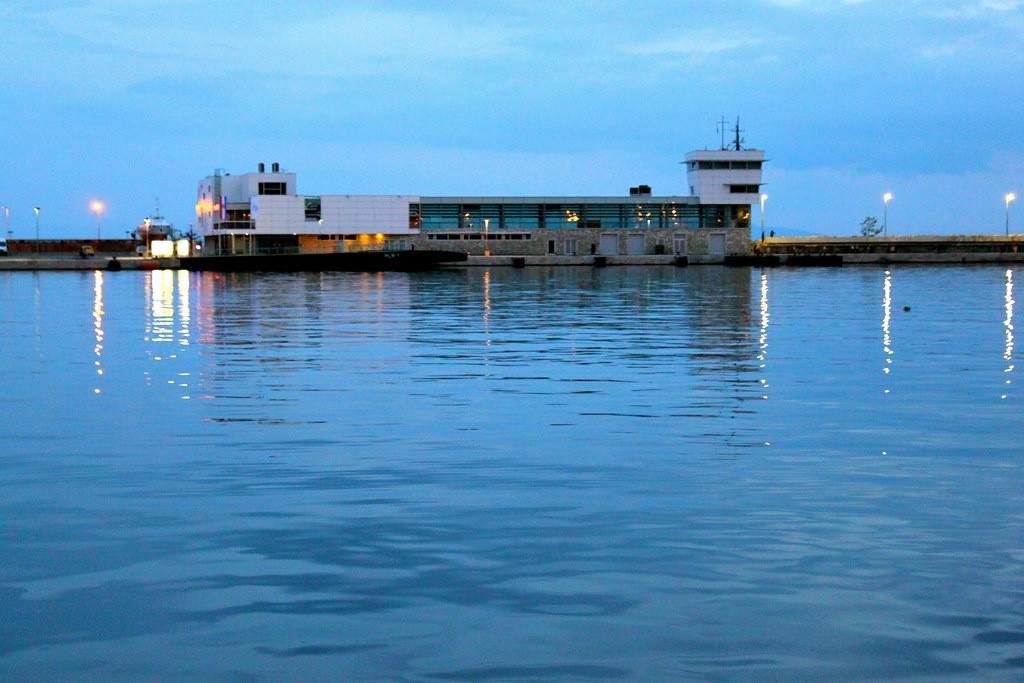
136;245;151;257
80;245;95;257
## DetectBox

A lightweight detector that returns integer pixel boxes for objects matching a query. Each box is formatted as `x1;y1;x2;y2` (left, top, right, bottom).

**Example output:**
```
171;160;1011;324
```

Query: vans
0;238;9;256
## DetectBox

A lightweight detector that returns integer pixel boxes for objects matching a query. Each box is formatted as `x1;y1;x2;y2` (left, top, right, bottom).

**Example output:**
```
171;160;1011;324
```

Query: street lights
1005;193;1015;235
760;194;768;242
883;193;892;237
145;217;151;258
33;206;42;252
93;203;100;251
